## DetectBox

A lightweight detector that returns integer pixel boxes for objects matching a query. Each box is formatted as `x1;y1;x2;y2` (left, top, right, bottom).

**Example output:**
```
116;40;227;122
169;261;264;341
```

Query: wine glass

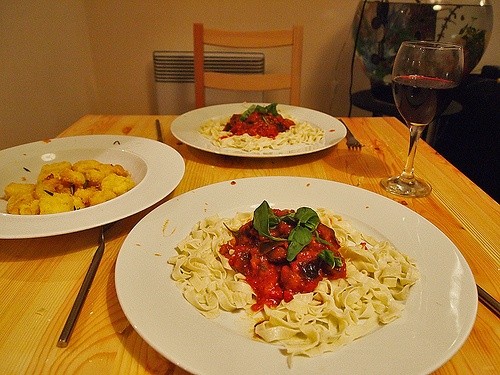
380;41;465;198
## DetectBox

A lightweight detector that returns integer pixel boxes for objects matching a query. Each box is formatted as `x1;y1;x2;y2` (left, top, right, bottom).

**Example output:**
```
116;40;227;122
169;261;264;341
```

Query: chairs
194;22;304;109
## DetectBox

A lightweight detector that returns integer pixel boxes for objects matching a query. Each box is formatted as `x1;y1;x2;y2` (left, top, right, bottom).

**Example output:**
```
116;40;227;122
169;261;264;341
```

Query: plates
170;102;347;158
0;135;186;239
115;176;479;375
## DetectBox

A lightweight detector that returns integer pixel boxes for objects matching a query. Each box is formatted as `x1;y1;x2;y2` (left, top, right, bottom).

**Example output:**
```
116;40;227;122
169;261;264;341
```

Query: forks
338;119;365;147
57;220;118;348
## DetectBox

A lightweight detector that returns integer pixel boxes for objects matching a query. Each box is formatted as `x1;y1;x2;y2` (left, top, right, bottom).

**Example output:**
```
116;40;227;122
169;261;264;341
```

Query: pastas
198;101;325;153
167;198;418;357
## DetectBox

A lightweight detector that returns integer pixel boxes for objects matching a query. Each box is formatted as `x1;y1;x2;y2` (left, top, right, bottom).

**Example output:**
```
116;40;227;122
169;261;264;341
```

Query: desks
352;87;471;154
0;113;500;375
